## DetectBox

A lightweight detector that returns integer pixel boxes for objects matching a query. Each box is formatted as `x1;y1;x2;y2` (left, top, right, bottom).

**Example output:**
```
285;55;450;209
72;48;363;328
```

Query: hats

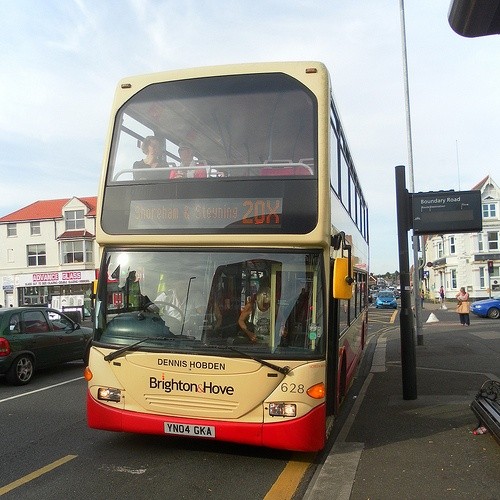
141;136;161;155
177;142;193;153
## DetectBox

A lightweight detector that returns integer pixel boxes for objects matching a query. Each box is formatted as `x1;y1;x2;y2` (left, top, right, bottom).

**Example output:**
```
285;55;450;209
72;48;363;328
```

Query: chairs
167;160;225;178
263;158;314;176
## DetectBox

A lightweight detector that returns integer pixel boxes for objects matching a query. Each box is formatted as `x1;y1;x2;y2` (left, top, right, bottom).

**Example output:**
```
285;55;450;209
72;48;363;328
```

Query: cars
376;290;397;309
369;285;401;304
470;294;500;318
0;307;93;384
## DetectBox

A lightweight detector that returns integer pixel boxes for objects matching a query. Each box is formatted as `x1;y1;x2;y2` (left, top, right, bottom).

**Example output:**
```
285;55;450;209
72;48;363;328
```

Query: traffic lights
488;261;493;273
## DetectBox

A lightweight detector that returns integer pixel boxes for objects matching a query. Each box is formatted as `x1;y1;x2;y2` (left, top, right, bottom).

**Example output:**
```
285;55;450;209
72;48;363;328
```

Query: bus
86;63;369;457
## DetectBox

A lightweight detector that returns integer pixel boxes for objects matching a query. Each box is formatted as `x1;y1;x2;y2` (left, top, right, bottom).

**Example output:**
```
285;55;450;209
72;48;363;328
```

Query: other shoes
460;324;464;325
465;324;468;327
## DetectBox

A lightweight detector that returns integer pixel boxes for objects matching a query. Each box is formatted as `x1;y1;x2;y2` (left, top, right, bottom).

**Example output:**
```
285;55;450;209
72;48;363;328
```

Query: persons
170;142;207;178
456;287;471;327
133;136;169;179
239;287;288;344
440;286;444;299
147;273;210;340
421;293;424;308
